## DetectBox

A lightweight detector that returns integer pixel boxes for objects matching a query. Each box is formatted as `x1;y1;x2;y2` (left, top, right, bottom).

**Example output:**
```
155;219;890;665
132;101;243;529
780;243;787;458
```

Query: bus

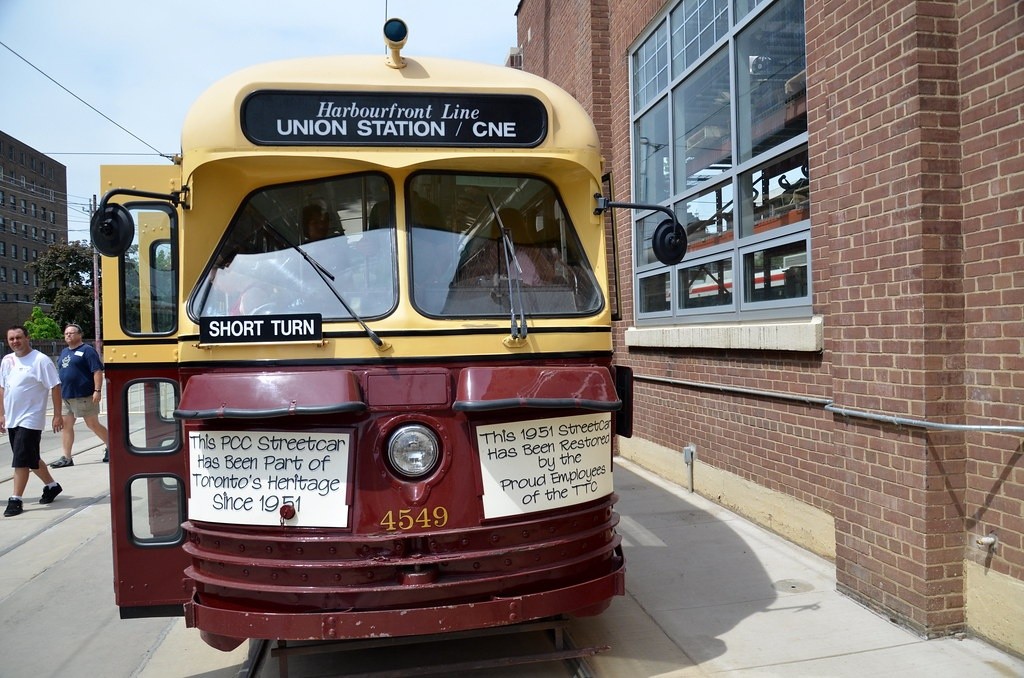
85;17;687;654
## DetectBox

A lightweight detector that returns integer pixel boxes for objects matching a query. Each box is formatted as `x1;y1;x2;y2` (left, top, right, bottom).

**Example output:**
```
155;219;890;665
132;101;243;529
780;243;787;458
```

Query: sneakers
103;448;110;462
50;456;74;467
39;483;63;504
4;497;23;516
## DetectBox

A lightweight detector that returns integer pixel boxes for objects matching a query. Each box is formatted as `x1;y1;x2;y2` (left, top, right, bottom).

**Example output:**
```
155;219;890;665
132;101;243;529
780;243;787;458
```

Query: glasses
64;332;81;335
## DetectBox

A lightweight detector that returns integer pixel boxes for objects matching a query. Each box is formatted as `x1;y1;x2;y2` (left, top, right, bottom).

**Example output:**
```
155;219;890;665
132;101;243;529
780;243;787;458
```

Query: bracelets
94;390;101;392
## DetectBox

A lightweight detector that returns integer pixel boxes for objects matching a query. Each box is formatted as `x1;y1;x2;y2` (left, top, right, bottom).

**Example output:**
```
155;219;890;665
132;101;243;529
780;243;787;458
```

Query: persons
0;326;64;516
47;323;113;467
199;170;586;330
